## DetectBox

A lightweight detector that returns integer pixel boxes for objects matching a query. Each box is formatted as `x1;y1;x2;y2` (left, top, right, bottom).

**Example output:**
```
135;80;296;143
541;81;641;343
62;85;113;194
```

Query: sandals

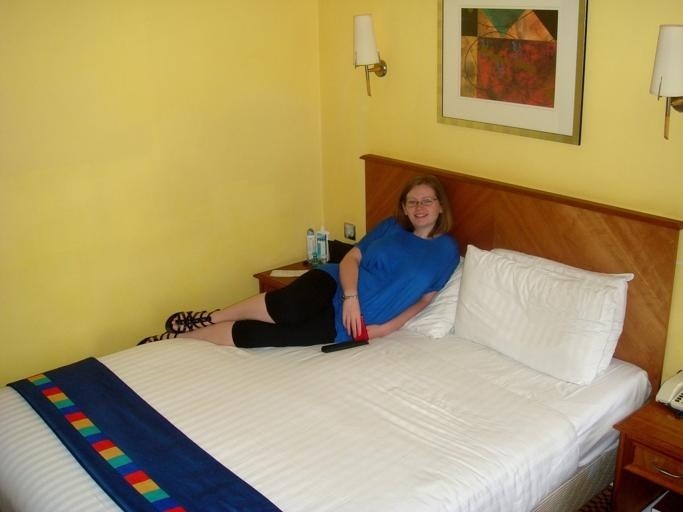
164;309;220;333
134;331;177;346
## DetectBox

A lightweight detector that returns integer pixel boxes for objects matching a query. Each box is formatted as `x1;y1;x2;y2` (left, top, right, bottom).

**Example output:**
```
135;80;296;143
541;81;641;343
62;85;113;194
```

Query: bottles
307;223;329;264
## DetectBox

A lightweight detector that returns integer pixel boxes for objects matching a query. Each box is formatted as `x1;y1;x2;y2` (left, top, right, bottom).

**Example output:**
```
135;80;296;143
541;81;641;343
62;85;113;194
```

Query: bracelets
342;294;358;299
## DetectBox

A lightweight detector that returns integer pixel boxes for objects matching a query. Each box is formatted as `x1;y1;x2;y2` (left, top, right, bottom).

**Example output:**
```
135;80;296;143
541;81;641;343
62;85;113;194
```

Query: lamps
650;24;683;140
353;14;387;96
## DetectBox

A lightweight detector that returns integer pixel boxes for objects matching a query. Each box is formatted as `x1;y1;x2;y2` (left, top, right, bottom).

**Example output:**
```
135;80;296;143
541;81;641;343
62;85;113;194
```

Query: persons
135;173;462;348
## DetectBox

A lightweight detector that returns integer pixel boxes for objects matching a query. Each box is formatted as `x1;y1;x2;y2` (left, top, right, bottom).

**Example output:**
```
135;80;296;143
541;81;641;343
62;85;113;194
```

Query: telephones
654;370;682;411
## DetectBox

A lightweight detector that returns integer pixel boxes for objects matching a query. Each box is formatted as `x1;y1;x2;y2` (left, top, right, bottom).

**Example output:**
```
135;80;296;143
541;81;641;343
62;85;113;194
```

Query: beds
0;155;683;512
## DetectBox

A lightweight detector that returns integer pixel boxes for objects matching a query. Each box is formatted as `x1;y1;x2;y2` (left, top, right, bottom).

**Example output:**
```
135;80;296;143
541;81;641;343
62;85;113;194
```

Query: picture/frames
437;0;588;146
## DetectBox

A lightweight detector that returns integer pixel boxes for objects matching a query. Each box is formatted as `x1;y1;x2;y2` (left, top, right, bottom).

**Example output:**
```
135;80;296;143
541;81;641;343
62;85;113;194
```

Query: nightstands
253;258;312;295
613;398;683;511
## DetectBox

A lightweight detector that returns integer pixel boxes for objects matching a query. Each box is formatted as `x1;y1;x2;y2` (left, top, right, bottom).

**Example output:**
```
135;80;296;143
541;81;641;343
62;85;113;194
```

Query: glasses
404;196;440;208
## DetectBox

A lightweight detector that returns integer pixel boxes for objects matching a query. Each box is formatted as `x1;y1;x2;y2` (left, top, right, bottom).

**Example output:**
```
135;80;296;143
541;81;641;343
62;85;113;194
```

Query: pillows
492;247;633;372
455;243;617;387
402;257;465;340
